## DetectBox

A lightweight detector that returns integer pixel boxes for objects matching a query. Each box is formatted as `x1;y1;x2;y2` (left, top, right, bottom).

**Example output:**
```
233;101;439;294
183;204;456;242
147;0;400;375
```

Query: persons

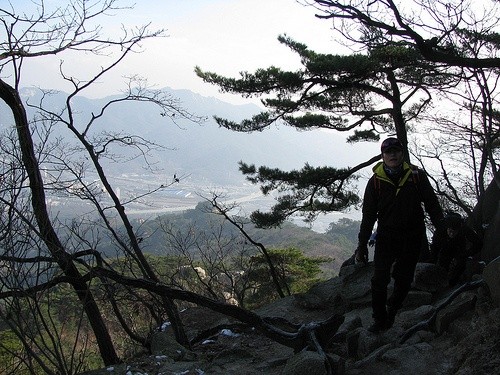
338;138;485;336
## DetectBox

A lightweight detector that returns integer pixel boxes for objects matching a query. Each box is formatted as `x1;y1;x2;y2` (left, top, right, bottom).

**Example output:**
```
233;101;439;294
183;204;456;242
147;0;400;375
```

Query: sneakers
366;312;387;332
386;296;403;328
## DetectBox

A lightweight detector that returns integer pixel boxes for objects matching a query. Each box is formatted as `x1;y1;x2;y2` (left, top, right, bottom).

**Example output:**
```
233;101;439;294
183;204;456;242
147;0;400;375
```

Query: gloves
354;242;369;265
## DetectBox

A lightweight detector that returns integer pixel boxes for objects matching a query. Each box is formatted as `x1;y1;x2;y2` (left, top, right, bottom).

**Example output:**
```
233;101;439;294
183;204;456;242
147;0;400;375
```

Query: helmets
381;138;404;153
